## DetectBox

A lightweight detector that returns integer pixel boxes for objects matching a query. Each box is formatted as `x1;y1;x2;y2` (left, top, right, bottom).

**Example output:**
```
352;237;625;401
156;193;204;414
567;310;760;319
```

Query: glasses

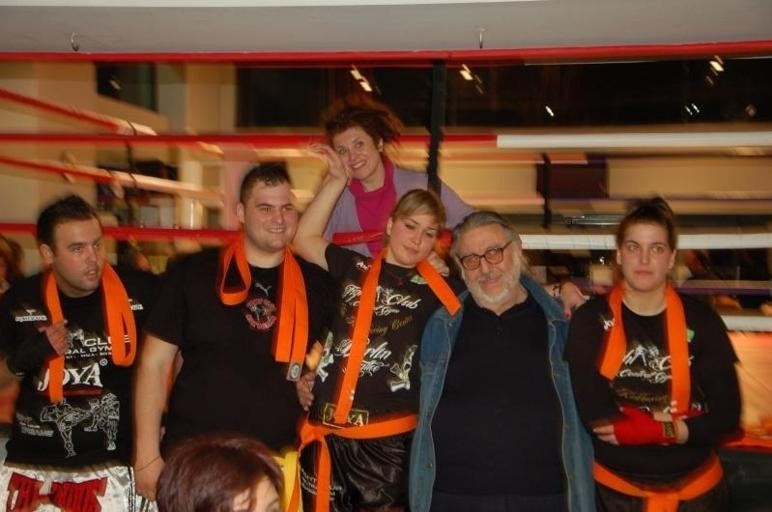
455;239;513;271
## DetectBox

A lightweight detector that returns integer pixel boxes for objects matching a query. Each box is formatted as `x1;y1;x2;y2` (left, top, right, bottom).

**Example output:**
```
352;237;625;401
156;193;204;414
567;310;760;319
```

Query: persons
314;96;479;264
158;429;290;512
565;191;745;512
288;138;589;512
3;194;158;509
134;160;343;504
297;203;598;512
2;232;770;342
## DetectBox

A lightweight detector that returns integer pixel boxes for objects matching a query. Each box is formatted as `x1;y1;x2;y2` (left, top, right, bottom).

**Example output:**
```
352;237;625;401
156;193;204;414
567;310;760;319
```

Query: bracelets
550;279;569;296
